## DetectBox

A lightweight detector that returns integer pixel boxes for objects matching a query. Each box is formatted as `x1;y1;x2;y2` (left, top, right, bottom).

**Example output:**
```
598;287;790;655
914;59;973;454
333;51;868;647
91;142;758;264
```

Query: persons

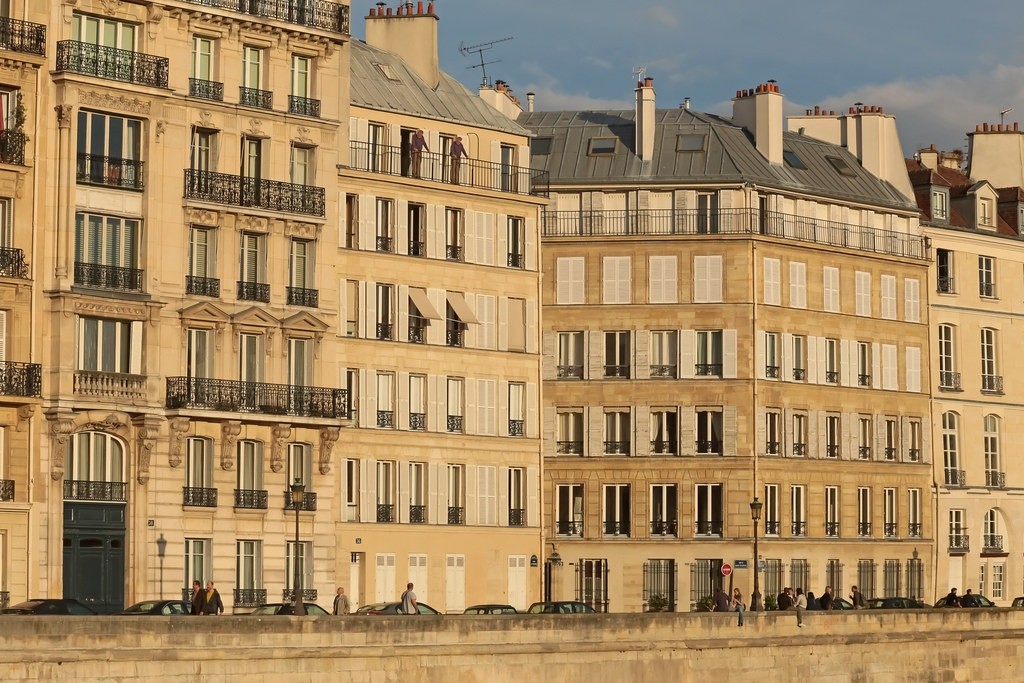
713;586;731;612
733;587;744;611
962;589;979;607
401;583;418;614
204;581;224;616
820;586;834;610
946;588;963;608
778;587;816;611
450;136;469;184
190;581;207;615
411;130;430;179
849;586;862;606
332;587;350;615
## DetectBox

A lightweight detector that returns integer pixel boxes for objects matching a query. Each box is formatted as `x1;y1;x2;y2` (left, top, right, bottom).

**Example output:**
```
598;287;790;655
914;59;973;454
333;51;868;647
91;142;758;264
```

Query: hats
717;587;725;591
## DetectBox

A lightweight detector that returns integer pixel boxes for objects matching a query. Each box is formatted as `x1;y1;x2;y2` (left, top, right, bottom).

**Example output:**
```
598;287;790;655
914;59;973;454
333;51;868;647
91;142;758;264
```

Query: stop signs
721;564;732;576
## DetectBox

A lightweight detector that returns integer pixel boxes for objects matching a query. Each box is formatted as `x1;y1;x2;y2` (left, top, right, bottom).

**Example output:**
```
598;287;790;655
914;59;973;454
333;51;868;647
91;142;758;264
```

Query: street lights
749;496;764;611
290;475;308;616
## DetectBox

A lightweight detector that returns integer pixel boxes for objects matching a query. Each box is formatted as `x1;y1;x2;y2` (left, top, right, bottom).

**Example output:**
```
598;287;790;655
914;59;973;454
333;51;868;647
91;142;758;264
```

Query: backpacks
859;593;868;606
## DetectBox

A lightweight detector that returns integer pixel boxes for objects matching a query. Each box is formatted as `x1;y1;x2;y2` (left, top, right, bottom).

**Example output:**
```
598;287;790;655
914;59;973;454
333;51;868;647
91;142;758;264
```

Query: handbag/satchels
418;610;420;614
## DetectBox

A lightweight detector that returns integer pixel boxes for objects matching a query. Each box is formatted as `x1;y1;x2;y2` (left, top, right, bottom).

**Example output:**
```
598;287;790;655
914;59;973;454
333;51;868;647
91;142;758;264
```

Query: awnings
446;291;481;325
409;288;443;320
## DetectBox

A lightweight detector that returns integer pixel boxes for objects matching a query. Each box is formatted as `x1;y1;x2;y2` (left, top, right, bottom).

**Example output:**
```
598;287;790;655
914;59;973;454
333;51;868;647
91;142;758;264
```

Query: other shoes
799;624;806;626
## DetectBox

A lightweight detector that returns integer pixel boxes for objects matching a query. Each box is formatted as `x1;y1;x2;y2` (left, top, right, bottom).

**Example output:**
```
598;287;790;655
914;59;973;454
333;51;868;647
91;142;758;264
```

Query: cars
527;600;598;613
462;604;519;614
352;602;443;615
248;603;332;616
0;597;101;616
866;597;925;609
933;594;999;608
815;598;854;610
1011;597;1024;608
109;598;192;615
